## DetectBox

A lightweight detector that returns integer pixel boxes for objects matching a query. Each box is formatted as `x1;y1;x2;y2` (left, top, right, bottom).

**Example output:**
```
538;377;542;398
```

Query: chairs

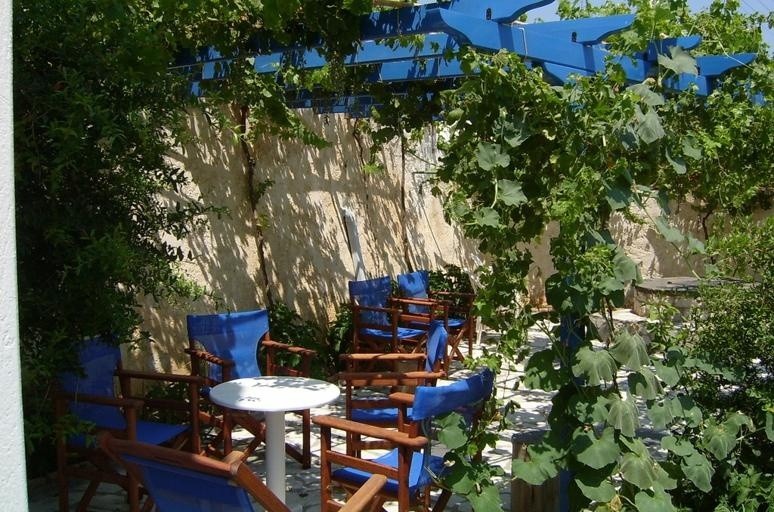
309;366;490;508
338;275;439;377
92;428;294;511
396;272;481;381
53;320;211;512
182;307;316;473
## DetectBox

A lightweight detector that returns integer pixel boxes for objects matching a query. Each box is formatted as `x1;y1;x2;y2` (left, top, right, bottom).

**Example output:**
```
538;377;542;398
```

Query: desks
202;374;344;510
